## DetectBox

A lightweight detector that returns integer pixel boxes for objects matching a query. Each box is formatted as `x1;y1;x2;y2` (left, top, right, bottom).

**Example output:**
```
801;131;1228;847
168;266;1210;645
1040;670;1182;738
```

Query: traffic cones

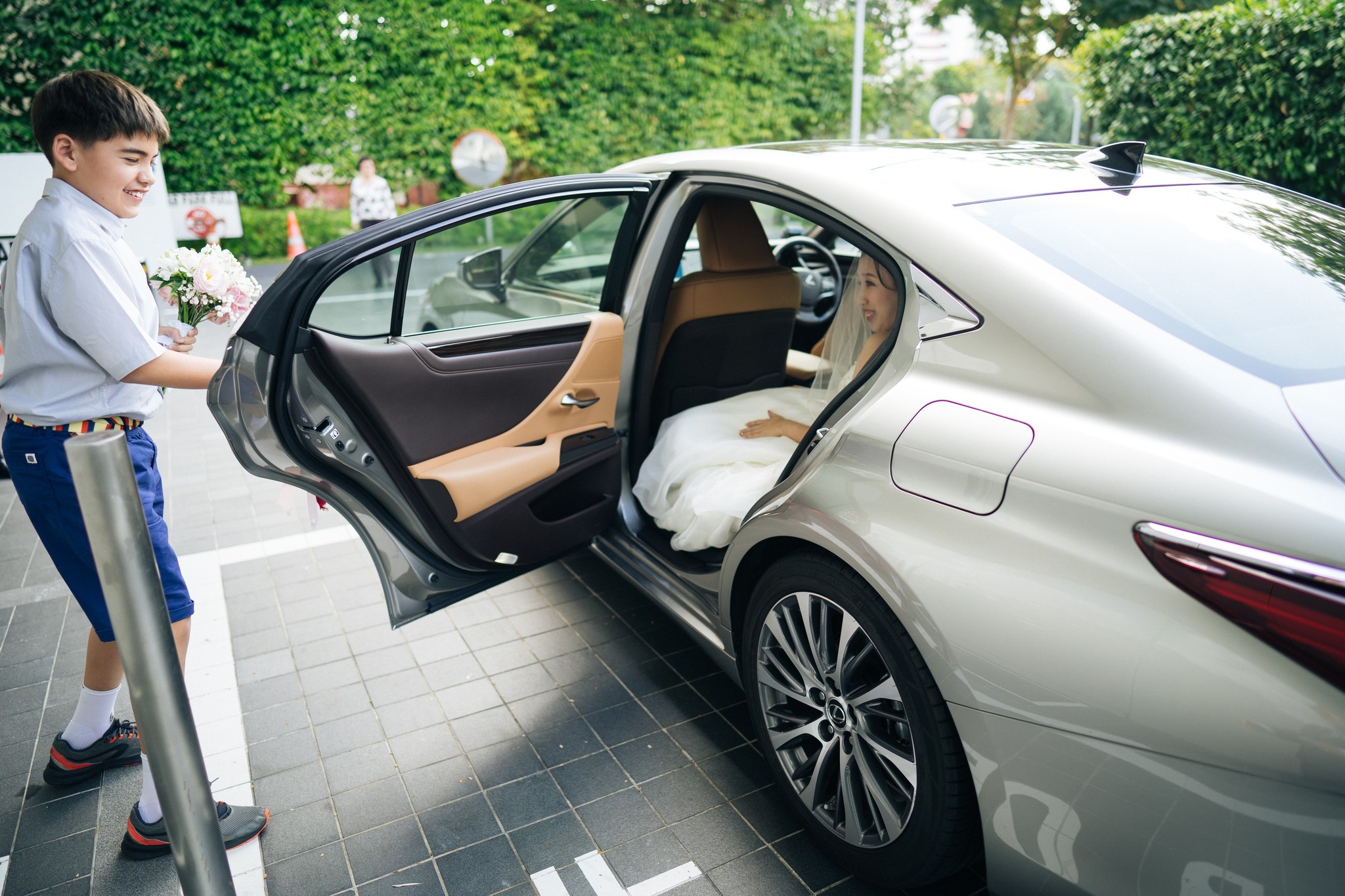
282;211;309;260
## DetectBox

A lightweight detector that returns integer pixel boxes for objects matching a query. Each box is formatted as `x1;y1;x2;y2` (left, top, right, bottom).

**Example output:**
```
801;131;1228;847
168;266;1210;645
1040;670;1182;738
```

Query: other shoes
373;281;384;290
386;276;396;289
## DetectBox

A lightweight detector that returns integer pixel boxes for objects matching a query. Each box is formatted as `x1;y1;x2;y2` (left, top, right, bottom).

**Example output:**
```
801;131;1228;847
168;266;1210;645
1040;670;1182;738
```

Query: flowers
141;242;265;400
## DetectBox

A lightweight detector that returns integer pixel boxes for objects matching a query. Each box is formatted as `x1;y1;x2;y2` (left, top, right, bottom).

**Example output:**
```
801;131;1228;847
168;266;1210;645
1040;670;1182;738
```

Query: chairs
652;191;804;434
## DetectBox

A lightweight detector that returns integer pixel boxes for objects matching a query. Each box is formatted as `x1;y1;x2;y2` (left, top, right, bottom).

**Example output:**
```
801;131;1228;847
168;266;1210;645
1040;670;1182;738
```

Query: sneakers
43;717;142;786
121;800;270;860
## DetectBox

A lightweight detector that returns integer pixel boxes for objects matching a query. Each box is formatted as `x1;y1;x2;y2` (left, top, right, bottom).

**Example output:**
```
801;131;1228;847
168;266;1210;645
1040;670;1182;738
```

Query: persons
351;157;398;290
0;72;271;860
630;253;901;554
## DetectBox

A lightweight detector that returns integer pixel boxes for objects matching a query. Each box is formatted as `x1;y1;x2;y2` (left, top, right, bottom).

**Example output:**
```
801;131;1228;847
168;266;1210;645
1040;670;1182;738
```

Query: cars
207;137;1345;896
